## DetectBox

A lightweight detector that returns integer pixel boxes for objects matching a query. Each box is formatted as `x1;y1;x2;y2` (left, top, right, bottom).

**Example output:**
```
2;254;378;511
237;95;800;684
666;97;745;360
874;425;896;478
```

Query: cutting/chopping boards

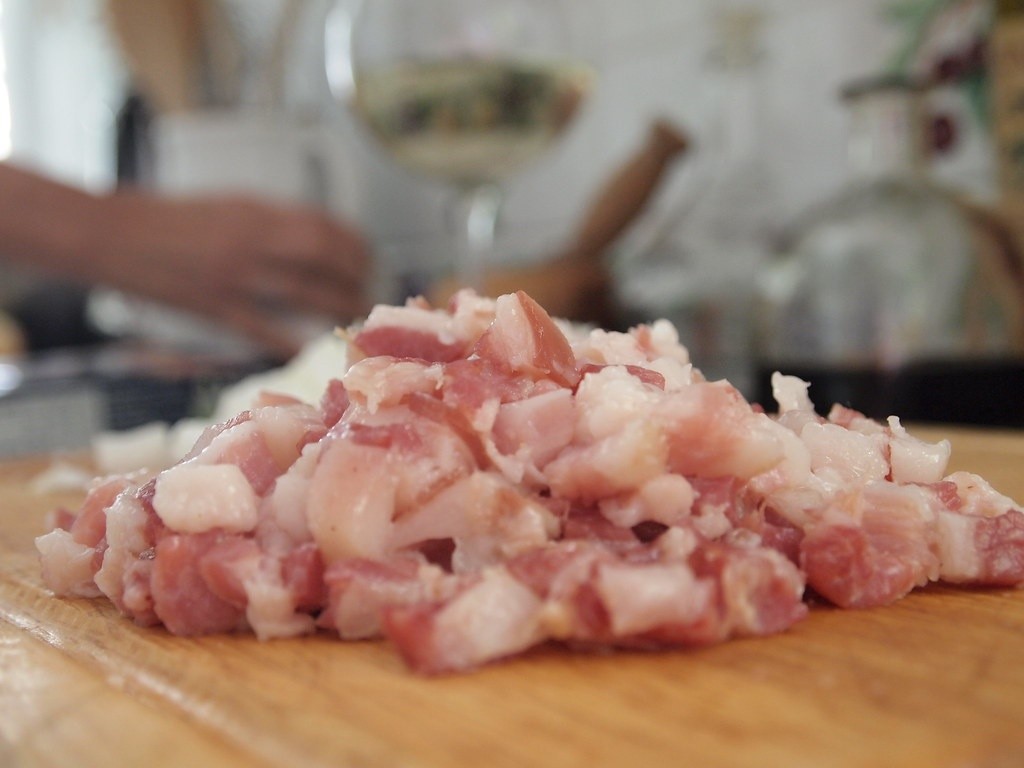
0;423;1024;768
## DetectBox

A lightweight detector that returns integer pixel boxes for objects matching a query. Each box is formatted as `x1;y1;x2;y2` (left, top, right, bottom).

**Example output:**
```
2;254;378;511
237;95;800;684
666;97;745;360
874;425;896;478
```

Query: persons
1;161;368;351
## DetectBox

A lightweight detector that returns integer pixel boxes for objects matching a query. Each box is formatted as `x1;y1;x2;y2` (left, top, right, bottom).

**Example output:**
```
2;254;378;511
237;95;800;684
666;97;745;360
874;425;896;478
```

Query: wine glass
328;1;598;312
